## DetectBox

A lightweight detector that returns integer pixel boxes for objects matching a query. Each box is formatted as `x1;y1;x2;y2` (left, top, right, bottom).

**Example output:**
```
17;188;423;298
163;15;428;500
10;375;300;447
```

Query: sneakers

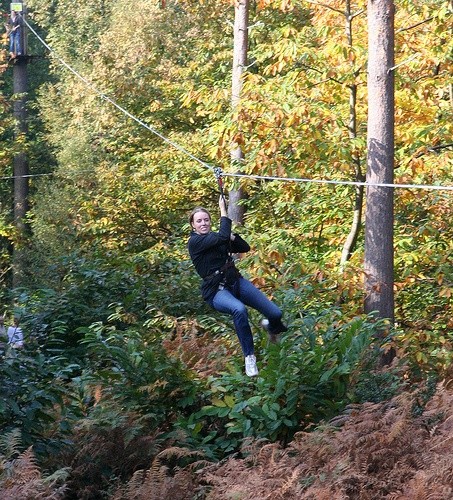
244;353;259;377
260;318;281;344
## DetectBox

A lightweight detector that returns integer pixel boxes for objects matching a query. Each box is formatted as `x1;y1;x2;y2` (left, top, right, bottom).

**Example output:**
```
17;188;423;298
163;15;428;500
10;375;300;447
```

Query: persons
0;317;26;353
188;195;285;377
5;10;22;55
